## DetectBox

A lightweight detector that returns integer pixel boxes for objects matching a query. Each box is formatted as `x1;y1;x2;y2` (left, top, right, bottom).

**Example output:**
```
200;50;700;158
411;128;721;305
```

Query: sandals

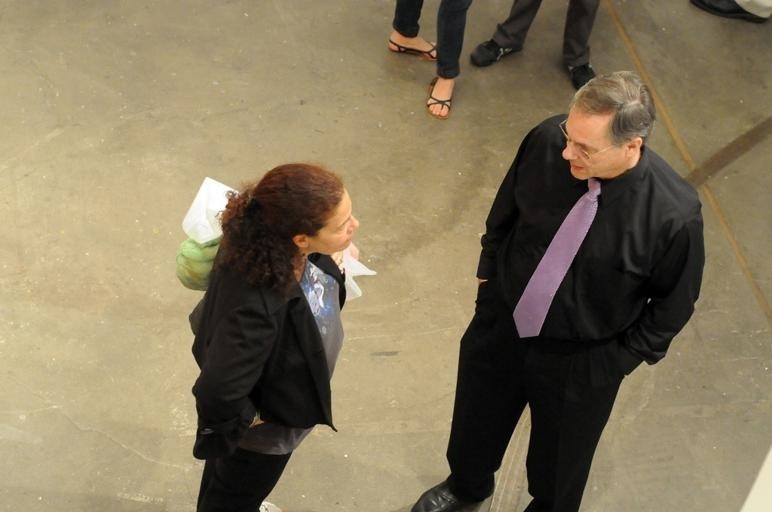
389;38;438;61
428;76;452;120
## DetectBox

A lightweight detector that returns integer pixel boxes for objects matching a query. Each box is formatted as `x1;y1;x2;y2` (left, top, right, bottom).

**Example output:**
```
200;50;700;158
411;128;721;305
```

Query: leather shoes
408;478;474;512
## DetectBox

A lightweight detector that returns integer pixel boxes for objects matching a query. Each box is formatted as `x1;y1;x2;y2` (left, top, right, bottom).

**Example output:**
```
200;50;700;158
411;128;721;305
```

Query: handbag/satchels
176;234;223;291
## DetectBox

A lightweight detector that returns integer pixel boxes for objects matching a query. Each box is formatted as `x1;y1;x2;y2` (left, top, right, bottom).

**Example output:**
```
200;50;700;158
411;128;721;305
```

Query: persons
184;163;361;510
402;70;706;511
471;0;601;92
383;1;473;119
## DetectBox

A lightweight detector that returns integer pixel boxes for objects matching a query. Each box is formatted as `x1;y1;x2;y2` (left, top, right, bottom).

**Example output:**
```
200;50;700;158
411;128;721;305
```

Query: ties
512;178;601;339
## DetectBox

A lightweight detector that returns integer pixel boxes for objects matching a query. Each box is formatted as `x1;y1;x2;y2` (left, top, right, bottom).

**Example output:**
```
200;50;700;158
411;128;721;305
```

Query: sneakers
259;501;283;511
470;37;517;67
564;59;596;90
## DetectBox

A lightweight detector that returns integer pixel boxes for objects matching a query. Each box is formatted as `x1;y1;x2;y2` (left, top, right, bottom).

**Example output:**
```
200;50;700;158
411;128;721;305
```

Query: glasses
559;118;624;159
